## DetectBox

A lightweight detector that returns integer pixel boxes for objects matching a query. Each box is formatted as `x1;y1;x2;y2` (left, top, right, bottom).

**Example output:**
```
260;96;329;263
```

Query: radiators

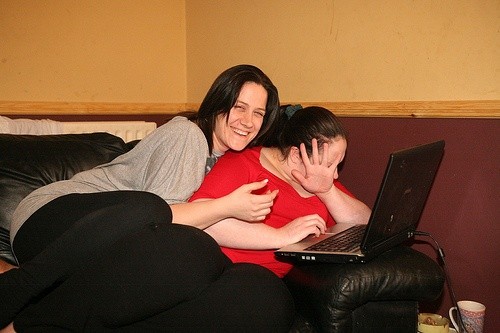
0;122;157;143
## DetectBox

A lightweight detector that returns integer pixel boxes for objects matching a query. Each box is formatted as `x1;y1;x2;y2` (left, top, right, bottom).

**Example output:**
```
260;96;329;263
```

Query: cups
449;301;486;333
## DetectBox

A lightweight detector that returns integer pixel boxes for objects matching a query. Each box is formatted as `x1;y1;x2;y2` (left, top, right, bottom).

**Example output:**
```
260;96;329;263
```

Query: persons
0;65;280;333
116;104;373;333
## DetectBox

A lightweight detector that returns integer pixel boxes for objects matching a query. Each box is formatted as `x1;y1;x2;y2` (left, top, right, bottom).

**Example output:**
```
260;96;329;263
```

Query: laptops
277;138;446;261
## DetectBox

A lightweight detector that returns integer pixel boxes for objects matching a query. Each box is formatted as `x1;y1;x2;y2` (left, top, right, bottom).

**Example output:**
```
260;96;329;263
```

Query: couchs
0;132;445;333
